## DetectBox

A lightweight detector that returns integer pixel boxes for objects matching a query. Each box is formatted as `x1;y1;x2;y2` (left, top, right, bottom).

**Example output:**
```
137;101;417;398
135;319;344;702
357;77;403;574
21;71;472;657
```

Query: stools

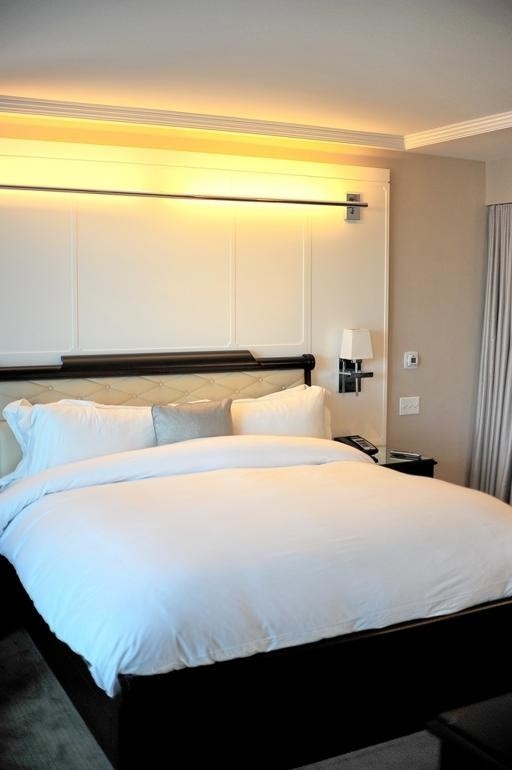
423;691;512;770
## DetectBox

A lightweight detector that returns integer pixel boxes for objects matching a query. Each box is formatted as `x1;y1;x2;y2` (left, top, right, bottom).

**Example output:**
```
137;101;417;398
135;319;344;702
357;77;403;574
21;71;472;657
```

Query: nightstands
369;446;437;478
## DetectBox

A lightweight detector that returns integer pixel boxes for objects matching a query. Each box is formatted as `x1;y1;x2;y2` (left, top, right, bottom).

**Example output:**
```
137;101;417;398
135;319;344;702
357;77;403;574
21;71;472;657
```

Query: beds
1;349;511;768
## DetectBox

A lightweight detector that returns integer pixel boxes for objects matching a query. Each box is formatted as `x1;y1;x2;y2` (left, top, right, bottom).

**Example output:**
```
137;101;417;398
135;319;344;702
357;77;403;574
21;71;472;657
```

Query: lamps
338;327;374;397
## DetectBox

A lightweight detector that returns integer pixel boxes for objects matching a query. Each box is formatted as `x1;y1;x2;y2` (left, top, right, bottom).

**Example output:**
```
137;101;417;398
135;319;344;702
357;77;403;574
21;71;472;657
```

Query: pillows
4;381;333;487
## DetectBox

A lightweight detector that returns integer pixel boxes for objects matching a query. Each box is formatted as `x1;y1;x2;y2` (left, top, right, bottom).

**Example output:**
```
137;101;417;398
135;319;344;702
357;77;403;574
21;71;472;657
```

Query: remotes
390;450;421;459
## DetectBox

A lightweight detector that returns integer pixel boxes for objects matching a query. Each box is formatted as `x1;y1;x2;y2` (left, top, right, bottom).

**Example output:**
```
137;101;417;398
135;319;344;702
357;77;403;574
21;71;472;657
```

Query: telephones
334;435;378;455
